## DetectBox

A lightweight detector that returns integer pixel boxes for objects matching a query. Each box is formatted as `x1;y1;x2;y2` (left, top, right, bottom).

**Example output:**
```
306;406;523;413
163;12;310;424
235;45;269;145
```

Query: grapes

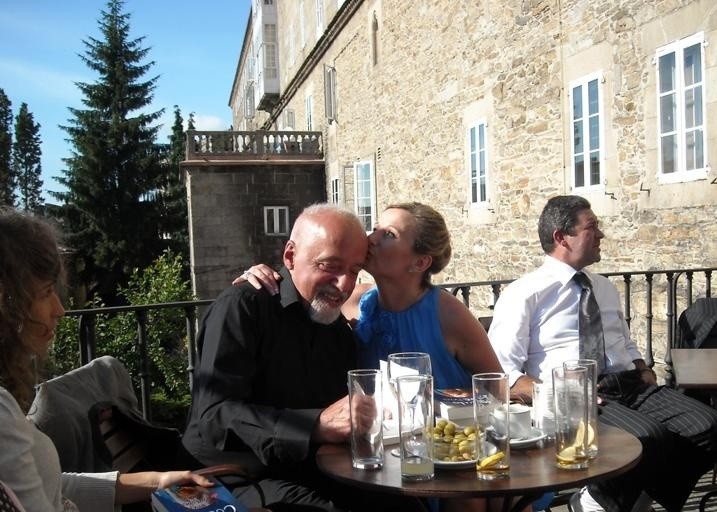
425;420;478;461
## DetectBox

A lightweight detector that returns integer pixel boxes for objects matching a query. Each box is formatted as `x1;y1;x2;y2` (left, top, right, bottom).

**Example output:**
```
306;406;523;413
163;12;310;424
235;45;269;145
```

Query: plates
486;423;549;449
361;419;422;445
417;437;498;471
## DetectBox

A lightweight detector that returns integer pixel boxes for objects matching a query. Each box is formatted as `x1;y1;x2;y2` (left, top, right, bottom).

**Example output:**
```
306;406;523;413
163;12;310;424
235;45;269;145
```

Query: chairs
32;356;265;512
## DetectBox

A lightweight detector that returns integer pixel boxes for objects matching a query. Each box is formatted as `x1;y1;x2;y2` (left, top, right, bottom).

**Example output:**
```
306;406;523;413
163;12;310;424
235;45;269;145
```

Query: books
430;385;501;421
431;412;491;432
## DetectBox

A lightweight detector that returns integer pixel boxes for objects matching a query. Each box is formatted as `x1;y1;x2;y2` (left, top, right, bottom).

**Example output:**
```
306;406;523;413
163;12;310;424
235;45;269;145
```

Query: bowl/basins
423;422;486;459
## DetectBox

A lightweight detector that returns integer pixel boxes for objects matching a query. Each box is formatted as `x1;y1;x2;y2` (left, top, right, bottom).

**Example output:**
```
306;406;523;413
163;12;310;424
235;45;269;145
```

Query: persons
486;194;716;512
181;202;431;511
663;295;716;411
0;202;271;512
231;202;555;512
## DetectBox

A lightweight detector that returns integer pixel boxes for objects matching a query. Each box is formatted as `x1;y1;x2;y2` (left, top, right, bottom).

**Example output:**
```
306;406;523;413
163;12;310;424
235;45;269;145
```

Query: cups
561;358;600;458
487;403;532;440
470;372;511;482
396;374;436;483
551;366;591;471
406;460;409;462
348;368;386;472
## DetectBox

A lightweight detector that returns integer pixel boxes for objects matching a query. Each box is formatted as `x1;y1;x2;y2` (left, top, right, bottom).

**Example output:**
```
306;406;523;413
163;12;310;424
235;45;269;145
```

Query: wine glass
384;350;432;457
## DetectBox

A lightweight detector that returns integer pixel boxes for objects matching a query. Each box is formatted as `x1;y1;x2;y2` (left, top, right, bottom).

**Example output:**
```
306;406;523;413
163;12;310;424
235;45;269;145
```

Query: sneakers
568;485;667;511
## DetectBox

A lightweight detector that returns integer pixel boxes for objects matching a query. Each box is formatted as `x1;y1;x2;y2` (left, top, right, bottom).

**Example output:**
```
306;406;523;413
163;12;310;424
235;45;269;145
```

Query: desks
669;347;717;511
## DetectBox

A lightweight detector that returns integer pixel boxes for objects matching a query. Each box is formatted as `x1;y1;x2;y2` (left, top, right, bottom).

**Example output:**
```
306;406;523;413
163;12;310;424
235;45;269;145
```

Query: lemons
573;421;594;447
557;447;576;463
479;452;505;469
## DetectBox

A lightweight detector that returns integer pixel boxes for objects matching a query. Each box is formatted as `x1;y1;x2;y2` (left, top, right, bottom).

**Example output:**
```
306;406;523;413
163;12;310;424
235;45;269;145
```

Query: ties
574;272;607;379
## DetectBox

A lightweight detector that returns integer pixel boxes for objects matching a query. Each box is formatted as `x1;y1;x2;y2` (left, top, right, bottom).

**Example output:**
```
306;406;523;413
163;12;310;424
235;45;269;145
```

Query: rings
243;270;252;278
